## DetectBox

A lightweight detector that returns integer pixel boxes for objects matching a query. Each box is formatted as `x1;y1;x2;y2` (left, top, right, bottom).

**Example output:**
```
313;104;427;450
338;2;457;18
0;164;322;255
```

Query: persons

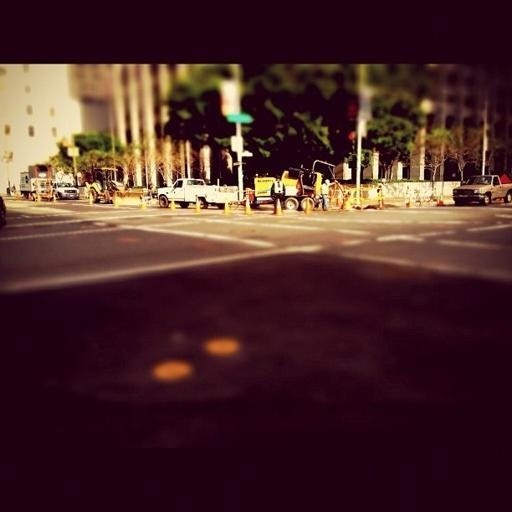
271;176;285;214
377;185;383;209
319;179;339;212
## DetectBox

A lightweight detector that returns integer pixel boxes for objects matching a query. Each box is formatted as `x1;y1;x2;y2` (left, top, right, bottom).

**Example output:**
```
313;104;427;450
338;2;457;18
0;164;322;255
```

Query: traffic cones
113;192;119;207
274;198;283;216
244;198;253;215
89;193;93;205
408;198;412;208
224;201;230;215
304;199;312;215
170;199;175;211
195;201;200;213
436;193;445;205
141;198;146;209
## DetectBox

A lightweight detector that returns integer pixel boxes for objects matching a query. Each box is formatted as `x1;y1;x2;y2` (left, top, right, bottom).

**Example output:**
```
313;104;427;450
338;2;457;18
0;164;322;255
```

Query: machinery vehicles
88;168;142;206
255;160;337;211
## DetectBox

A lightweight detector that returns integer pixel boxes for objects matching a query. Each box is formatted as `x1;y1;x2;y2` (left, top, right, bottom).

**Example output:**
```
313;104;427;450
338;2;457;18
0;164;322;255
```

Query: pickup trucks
158;178;238;208
453;175;512;205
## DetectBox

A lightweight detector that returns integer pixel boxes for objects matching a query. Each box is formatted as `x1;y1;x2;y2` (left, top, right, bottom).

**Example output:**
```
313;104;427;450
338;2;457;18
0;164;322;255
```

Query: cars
53;182;80;200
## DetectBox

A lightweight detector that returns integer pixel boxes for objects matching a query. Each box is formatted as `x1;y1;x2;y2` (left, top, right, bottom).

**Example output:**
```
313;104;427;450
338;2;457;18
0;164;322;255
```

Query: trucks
20;165;53;201
